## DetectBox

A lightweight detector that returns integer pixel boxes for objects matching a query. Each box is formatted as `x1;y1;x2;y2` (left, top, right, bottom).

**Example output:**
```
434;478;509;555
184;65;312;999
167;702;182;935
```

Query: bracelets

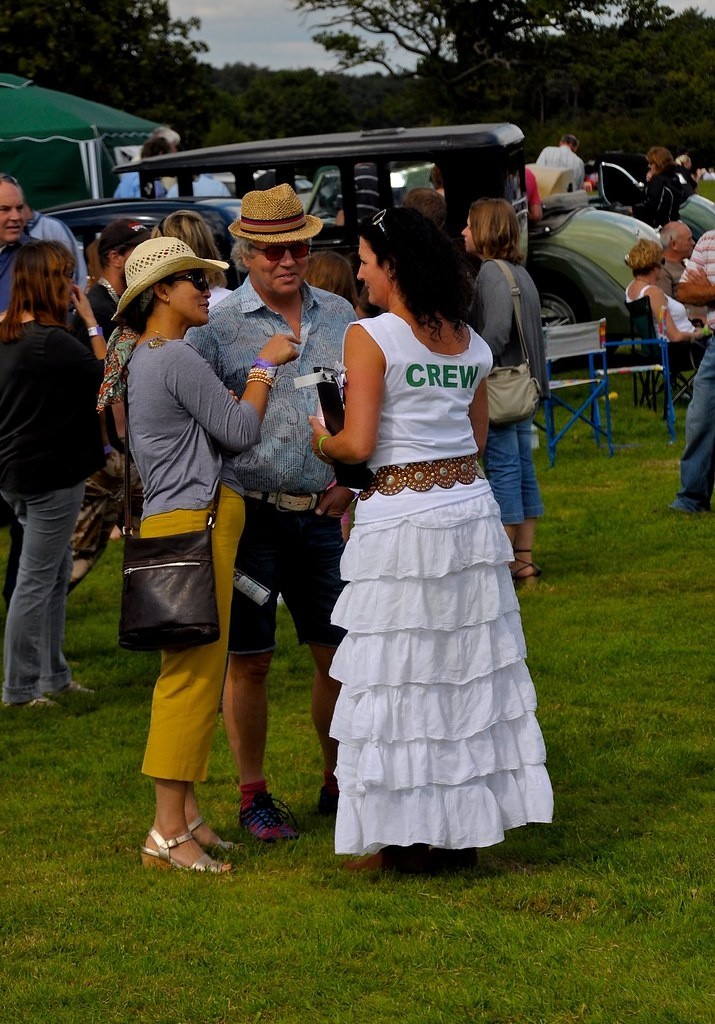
88;327;103;335
104;444;112;454
246;368;275;387
252;357;278;377
318;435;330;458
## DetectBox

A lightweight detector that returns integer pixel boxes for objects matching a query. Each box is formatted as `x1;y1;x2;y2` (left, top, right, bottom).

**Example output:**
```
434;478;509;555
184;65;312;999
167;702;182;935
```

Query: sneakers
240;793;298;840
316;787;342;816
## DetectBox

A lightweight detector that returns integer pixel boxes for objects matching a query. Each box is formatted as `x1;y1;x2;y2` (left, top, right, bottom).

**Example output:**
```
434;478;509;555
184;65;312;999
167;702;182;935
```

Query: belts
246;488;322;510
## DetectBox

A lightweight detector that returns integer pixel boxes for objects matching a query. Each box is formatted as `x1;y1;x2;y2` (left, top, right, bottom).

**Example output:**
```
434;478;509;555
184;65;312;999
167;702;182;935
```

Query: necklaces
96;276;120;305
143;329;169;339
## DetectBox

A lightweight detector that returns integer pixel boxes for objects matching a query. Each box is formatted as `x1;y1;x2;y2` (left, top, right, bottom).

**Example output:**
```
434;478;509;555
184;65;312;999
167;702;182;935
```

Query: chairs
531;294;713;469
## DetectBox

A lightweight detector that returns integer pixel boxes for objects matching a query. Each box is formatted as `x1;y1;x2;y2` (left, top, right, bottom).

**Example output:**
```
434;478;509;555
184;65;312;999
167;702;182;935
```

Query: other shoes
7;696;61;708
64;680;95;693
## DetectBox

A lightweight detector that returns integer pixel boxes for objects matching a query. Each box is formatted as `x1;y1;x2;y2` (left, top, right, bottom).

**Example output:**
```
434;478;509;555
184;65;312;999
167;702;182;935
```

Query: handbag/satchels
116;528;219;651
486;362;543;423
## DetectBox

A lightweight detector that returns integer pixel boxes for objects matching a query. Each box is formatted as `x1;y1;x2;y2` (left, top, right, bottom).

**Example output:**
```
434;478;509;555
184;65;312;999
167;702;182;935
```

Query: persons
305;187;555;874
621;240;707;342
183;185;366;840
92;240;300;877
0;126;715;716
462;197;546;581
672;229;715;515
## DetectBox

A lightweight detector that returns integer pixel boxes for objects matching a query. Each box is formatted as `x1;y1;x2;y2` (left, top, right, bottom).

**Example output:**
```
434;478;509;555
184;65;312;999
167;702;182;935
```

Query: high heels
188;818;249;855
141;829;232;875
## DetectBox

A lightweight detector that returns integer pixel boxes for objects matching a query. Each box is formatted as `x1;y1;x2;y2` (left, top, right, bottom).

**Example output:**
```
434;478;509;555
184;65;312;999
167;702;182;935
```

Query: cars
588;152;715;243
529;204;664;369
112;124;529;291
34;198;244;282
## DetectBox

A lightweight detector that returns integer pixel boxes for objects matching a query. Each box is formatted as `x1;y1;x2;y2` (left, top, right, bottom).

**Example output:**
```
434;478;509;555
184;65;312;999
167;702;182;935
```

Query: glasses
157;271;210;292
374;208;389;242
251;244;312;261
66;270;74;278
157;218;167;236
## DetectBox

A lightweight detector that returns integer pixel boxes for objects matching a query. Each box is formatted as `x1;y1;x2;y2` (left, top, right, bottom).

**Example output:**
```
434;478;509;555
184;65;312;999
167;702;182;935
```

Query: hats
228;183;323;242
111;237;229;322
98;219;151;253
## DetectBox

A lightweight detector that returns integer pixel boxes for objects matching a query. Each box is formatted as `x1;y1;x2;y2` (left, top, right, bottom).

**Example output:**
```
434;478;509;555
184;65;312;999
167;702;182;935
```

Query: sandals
512;549;535;578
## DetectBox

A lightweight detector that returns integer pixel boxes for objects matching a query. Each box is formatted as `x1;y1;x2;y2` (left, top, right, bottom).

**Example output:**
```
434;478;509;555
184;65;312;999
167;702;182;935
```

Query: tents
0;72;172;202
